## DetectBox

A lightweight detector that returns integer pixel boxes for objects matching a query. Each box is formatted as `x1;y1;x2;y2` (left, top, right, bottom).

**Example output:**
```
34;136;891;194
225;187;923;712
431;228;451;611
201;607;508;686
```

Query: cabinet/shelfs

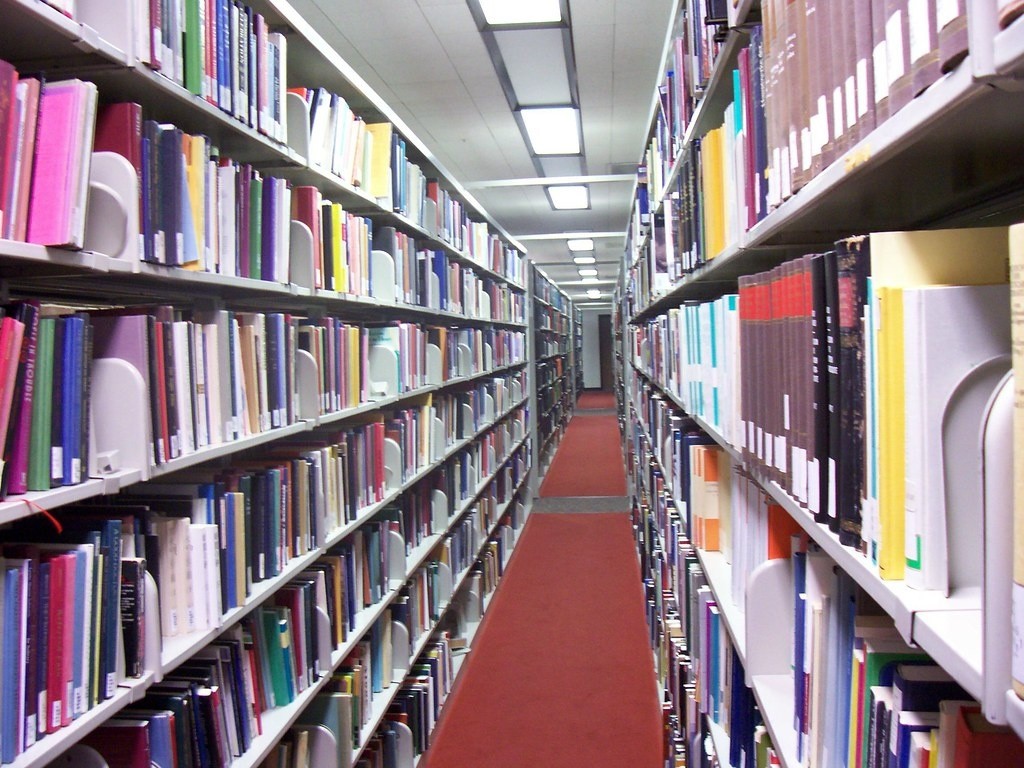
611;0;1024;768
0;0;583;768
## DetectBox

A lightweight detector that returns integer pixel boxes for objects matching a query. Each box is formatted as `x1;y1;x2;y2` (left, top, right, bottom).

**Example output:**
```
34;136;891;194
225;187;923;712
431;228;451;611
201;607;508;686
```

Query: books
609;0;1024;768
0;2;586;767
